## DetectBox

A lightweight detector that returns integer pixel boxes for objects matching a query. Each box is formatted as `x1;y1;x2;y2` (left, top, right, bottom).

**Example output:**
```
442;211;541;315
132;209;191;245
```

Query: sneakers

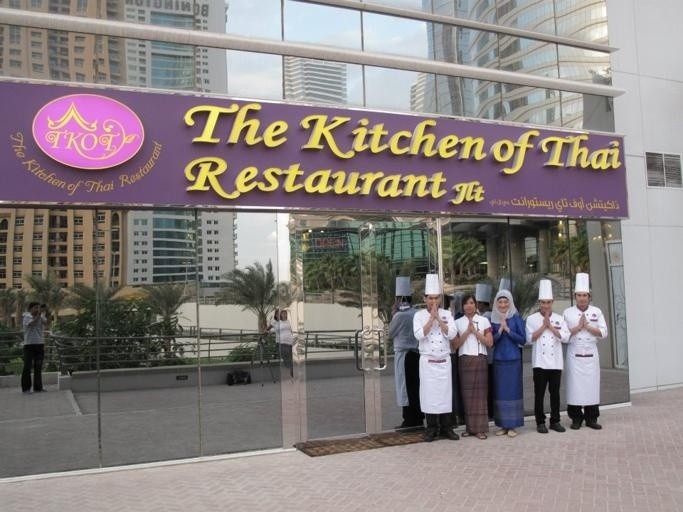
462;430;476;436
22;388;47;395
550;422;566;432
439;429;459;440
586;419;602;430
495;428;507;436
394;421;424;430
424;426;438;442
570;417;585;429
476;432;487;439
507;428;518;437
537;422;549;433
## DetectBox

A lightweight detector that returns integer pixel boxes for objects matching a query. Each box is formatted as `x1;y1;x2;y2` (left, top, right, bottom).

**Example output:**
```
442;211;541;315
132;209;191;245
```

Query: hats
476;284;492;302
538;279;554;302
499;278;515;290
575;272;590;293
395;276;412;297
424;273;442;295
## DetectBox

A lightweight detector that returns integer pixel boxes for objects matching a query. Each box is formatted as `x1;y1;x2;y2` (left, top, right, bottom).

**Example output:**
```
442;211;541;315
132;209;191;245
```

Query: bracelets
585;326;595;334
265;329;270;336
471;328;477;335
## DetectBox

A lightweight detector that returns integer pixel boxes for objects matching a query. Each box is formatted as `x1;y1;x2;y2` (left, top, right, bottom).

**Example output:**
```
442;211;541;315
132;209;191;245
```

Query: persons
263;310;279;348
561;290;608;429
21;301;54;396
525;299;571;433
412;294;458;440
387;294;493;433
280;309;295;379
451;295;494;440
491;290;527;437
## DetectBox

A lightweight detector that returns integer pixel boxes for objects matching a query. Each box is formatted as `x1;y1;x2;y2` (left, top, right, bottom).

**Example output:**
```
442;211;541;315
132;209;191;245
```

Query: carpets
294;429;441;457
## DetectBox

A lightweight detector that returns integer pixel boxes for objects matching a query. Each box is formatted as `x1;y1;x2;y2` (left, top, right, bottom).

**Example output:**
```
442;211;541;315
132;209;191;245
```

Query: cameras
39;303;47;308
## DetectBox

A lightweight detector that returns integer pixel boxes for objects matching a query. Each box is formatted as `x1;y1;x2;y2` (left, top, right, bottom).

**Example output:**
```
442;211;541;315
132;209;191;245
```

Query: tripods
244;339;276;387
